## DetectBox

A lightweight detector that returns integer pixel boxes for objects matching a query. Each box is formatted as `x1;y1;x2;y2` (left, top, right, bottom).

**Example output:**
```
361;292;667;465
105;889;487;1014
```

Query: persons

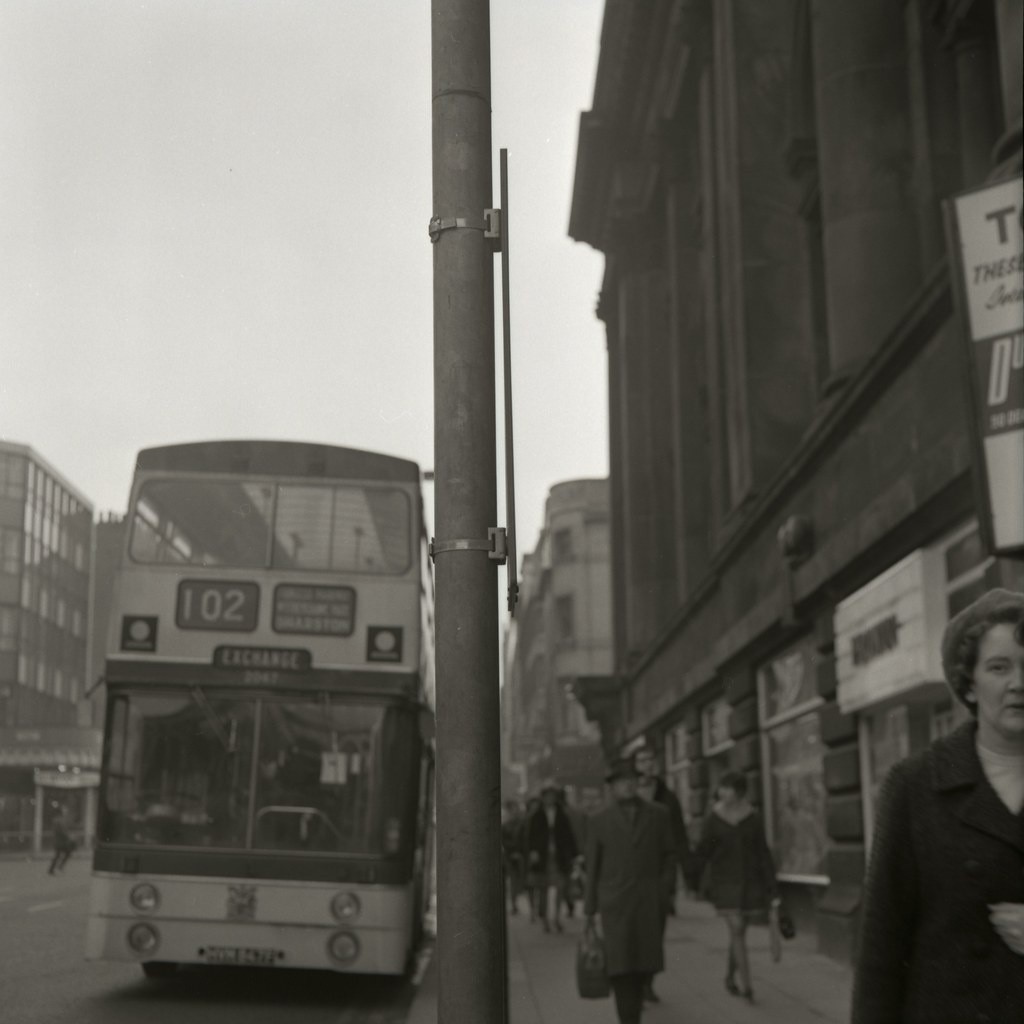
630;747;688;1005
49;802;72;875
698;769;781;1004
851;588;1024;1024
502;786;579;934
584;759;673;1024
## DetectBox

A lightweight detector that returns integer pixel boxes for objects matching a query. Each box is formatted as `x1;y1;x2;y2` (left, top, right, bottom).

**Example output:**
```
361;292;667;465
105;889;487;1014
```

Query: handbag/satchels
577;919;611;998
778;905;796;938
768;908;782;963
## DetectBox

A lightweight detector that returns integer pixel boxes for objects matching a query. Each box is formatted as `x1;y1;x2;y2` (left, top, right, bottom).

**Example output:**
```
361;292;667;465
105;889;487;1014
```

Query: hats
939;588;1024;718
604;757;644;784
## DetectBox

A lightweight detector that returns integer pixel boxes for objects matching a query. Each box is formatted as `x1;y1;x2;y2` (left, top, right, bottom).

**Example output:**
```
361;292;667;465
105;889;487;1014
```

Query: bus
87;436;437;983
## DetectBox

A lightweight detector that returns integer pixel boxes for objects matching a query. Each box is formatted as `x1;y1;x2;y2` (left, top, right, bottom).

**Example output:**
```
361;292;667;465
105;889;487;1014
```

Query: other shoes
58;864;65;873
725;976;741;997
644;975;660;1004
744;987;752;1001
47;870;55;877
668;904;675;916
